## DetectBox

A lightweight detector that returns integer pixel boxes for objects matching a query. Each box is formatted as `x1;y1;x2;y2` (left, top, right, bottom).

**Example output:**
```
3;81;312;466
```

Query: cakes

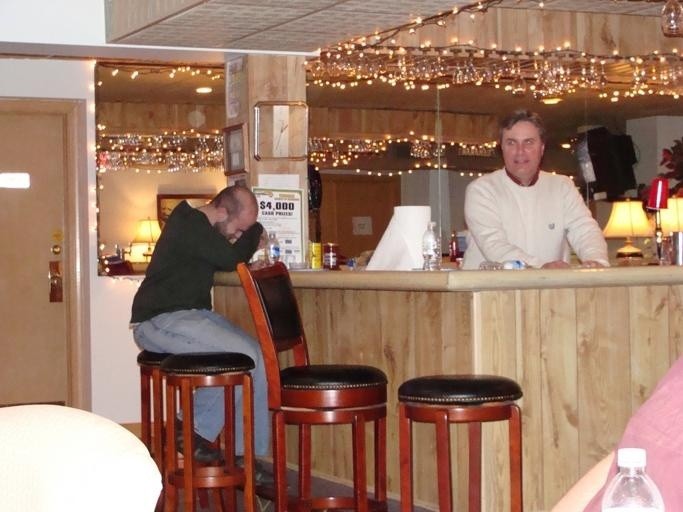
322;242;339;270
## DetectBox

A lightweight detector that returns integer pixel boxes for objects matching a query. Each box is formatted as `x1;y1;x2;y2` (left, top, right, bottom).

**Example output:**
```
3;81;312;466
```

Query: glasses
603;198;652;260
132;217;162;261
659;195;683;266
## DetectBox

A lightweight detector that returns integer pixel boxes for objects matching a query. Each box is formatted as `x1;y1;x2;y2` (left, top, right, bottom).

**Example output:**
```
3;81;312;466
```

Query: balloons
254;100;310;162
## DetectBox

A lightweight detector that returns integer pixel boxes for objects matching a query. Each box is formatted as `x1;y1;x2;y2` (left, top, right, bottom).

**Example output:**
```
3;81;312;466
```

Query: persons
126;183;276;485
459;110;611;269
547;353;683;512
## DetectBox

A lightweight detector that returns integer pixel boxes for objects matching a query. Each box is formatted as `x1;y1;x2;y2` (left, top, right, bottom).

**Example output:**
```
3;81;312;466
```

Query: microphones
165;351;256;512
137;349;224;512
397;374;523;512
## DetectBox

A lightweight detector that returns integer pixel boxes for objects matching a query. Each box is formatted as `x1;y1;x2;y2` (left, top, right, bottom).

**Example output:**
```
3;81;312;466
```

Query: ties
575;127;637;198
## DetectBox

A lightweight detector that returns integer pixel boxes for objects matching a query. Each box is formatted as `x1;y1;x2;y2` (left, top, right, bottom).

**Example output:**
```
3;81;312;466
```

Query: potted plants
157;194;218;231
222;122;249;176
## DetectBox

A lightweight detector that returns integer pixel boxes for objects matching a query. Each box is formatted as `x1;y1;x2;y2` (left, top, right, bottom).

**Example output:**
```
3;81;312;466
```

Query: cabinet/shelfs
323;242;340;271
266;231;279;269
308;242;321;269
421;221;441;271
282;240;301;263
478;257;529;271
447;228;458;263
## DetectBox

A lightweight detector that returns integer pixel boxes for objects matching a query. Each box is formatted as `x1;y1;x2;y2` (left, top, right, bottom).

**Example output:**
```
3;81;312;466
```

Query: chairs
162;421;220;461
237;456;275;485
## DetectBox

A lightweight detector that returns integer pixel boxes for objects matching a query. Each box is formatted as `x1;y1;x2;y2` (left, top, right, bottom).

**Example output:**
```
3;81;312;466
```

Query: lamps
237;260;388;512
